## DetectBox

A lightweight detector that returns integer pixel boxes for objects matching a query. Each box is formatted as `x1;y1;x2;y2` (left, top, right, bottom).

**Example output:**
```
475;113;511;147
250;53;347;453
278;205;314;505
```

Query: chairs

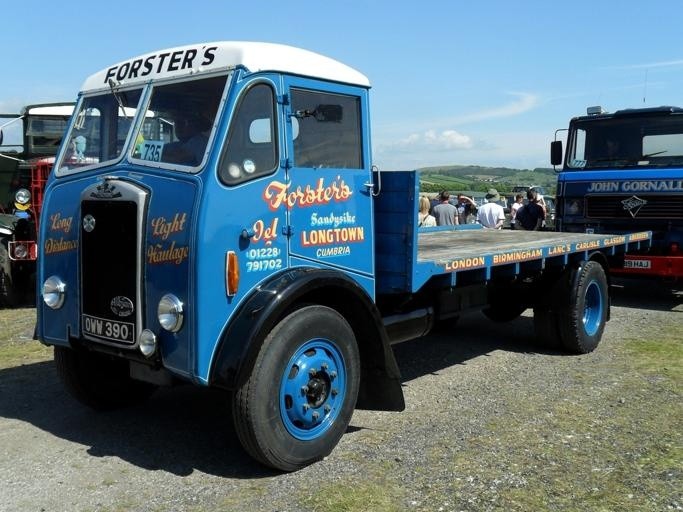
162;114;211;165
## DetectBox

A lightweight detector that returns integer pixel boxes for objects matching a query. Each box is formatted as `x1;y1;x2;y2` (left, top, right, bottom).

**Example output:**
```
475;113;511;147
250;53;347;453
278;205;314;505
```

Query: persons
418;188;546;231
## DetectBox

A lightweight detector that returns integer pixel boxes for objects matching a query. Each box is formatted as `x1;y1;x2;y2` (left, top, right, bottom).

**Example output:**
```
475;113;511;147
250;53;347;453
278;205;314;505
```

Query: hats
486;189;500;202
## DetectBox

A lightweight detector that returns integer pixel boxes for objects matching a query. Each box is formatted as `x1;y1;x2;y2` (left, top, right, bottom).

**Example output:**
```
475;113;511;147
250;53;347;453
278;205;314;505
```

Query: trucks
548;101;683;302
32;42;656;473
0;101;165;306
